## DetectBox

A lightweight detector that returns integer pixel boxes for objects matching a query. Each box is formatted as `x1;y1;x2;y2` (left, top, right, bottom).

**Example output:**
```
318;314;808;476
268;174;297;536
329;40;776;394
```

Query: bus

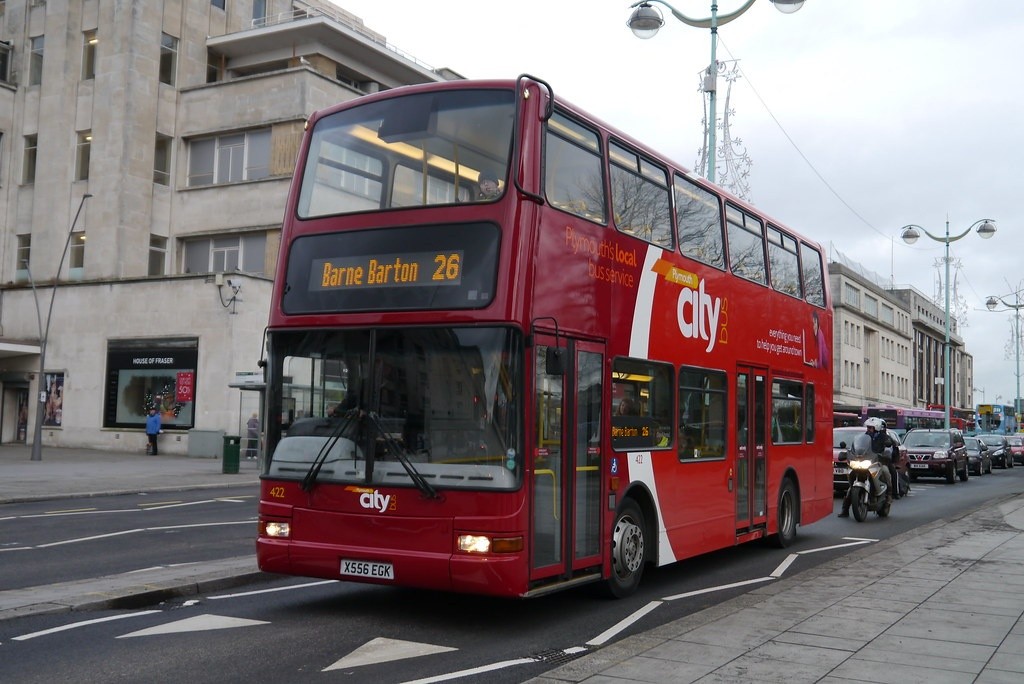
928;404;976;423
975;403;1016;437
949;417;975;433
833;412;862;429
862;404;945;434
256;74;836;603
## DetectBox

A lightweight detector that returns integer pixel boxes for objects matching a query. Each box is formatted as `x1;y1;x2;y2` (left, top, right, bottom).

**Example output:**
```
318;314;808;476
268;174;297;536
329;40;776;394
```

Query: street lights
984;295;1024;432
20;194;93;462
626;0;804;183
899;213;997;427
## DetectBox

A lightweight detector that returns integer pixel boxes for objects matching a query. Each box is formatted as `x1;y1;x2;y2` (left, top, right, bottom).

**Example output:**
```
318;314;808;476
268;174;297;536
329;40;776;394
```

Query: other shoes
837;512;849;517
253;456;257;460
246;457;251;460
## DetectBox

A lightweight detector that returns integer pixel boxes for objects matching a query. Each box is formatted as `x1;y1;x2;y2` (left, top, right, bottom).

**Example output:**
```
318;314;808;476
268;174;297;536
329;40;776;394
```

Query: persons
474;166;501;202
811;310;829;370
838;417;900;518
145;405;161;456
614;399;635;414
46;375;61;421
246;414;259;460
327;355;400;418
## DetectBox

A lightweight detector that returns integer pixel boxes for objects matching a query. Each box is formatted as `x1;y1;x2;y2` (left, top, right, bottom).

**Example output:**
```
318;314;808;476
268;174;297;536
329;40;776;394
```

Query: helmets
863;416;882;431
879;418;887;432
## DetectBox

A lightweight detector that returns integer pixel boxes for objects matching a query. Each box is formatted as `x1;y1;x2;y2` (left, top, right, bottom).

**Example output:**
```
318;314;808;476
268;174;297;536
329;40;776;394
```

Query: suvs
832;427;907;498
900;428;970;484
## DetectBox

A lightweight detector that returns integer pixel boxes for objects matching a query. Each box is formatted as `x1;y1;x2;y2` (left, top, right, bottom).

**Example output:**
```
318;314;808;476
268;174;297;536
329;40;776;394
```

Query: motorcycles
850;430;904;522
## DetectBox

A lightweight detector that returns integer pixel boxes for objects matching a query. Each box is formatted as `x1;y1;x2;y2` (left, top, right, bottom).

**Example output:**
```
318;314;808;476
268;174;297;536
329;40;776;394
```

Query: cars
962;437;992;475
975;434;1014;469
1006;436;1024;464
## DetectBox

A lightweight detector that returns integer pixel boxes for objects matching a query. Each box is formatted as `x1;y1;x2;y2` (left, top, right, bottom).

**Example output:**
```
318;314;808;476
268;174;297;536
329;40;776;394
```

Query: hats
149;407;156;410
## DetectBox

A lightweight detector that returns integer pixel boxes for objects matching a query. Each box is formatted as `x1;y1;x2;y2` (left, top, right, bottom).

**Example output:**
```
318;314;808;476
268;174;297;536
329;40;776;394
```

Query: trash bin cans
222;435;242;474
